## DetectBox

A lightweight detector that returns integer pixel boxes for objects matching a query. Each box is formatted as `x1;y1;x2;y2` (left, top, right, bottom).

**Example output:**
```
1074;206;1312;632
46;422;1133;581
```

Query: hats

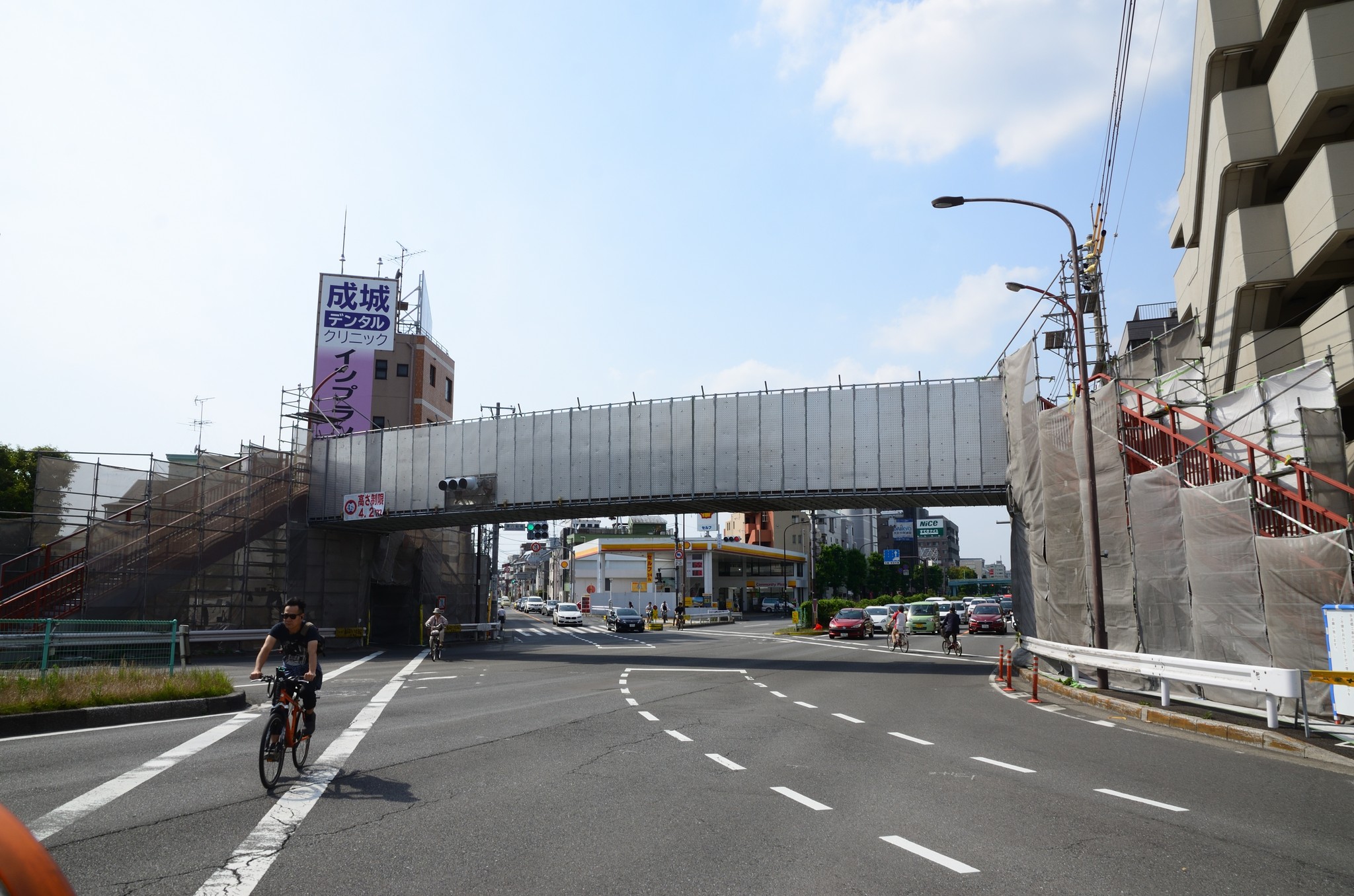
432;608;441;613
663;601;666;603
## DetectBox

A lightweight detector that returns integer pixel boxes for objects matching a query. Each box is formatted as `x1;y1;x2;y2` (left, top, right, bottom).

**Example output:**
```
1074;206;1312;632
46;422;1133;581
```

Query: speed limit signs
504;567;510;572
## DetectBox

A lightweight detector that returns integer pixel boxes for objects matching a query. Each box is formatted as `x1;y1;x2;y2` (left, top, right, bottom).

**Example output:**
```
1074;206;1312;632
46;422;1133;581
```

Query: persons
977;608;986;614
249;597;323;761
629;601;633;608
940;607;961;650
887;605;908;648
660;600;671;621
1010;608;1013;616
497;606;506;630
518;589;522;599
674;602;684;626
773;599;780;615
426;608;448;655
916;606;933;615
991;608;998;614
645;602;653;625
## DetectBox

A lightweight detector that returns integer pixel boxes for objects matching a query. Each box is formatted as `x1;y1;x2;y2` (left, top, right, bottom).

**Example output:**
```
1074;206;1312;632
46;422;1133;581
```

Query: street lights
930;193;1111;691
783;520;809;616
1005;282;1081;376
981;571;994;578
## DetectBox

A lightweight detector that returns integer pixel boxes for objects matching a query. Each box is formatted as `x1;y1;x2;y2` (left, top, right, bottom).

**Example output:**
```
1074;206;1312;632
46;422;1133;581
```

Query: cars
606;608;645;633
884;594;1014;626
968;603;1008;635
498;596;546;614
541;600;562;616
553;602;583;627
828;608;875;640
865;606;895;634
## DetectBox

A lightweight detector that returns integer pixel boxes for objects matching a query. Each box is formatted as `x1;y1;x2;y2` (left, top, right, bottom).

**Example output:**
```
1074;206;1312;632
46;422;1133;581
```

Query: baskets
678;615;684;619
940;627;947;637
886;624;894;633
898;626;911;633
429;627;439;636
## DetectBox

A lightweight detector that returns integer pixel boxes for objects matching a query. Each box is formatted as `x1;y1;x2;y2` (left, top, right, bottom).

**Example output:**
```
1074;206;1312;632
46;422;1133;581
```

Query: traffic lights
439;476;478;492
513;580;519;583
527;523;549;540
723;535;742;543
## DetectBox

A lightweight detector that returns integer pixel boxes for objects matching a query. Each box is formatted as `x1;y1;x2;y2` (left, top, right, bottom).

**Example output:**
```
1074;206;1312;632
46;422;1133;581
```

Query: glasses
281;611;301;619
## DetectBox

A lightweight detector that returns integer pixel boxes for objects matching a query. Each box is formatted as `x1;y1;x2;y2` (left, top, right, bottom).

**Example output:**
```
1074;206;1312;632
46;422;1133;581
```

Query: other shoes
662;617;663;619
429;650;433;655
893;641;898;647
676;622;678;626
501;627;503;630
947;644;953;650
647;623;648;625
666;619;668;621
439;644;443;649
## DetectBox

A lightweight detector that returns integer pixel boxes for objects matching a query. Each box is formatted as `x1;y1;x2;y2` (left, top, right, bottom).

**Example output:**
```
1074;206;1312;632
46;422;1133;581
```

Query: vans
905;601;941;635
761;598;796;612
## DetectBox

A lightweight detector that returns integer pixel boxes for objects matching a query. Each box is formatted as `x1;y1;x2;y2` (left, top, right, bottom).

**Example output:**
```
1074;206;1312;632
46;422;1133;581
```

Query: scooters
1010;612;1018;632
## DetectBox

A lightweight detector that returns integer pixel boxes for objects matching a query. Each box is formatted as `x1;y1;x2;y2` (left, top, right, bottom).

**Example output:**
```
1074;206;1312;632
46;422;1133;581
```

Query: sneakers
304;710;316;734
267;742;279;762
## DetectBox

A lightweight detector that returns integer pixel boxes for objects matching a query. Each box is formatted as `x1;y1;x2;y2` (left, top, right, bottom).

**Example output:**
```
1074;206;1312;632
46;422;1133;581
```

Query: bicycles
250;666;320;790
426;622;442;662
648;612;684;631
885;624;909;653
939;625;962;657
